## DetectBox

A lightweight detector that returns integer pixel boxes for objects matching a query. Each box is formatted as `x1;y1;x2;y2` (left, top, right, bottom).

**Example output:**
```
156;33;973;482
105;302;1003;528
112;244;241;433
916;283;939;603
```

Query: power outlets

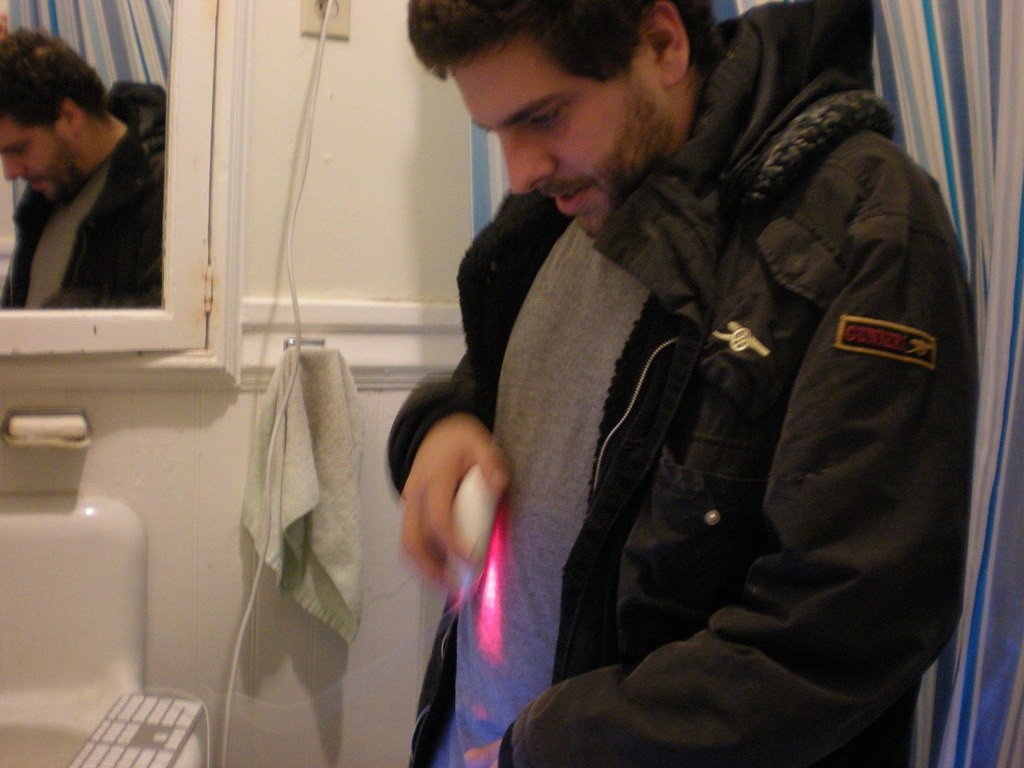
300;0;351;41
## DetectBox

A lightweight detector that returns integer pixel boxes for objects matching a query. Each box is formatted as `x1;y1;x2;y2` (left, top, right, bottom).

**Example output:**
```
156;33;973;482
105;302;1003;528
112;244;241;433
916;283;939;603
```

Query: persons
386;0;978;768
0;27;167;310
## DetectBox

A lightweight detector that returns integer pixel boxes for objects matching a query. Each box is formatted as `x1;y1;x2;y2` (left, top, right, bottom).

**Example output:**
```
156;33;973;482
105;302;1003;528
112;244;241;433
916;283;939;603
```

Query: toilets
0;486;204;768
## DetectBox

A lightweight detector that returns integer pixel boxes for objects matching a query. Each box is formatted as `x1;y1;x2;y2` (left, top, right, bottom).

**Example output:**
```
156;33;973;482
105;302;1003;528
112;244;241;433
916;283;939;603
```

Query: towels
234;344;370;647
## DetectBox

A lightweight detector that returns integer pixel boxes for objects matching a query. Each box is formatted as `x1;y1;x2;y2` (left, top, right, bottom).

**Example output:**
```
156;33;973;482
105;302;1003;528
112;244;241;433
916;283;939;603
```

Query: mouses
452;462;505;573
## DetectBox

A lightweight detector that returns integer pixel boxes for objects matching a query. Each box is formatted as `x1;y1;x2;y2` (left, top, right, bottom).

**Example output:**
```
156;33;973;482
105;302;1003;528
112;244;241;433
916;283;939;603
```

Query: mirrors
0;0;217;357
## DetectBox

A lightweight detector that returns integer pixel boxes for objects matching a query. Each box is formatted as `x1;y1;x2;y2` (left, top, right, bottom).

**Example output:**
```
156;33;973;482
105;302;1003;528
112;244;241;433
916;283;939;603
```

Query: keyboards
69;692;204;768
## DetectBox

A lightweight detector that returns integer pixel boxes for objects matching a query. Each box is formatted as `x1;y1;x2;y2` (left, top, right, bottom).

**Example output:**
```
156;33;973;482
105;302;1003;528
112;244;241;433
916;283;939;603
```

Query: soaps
8;412;88;442
